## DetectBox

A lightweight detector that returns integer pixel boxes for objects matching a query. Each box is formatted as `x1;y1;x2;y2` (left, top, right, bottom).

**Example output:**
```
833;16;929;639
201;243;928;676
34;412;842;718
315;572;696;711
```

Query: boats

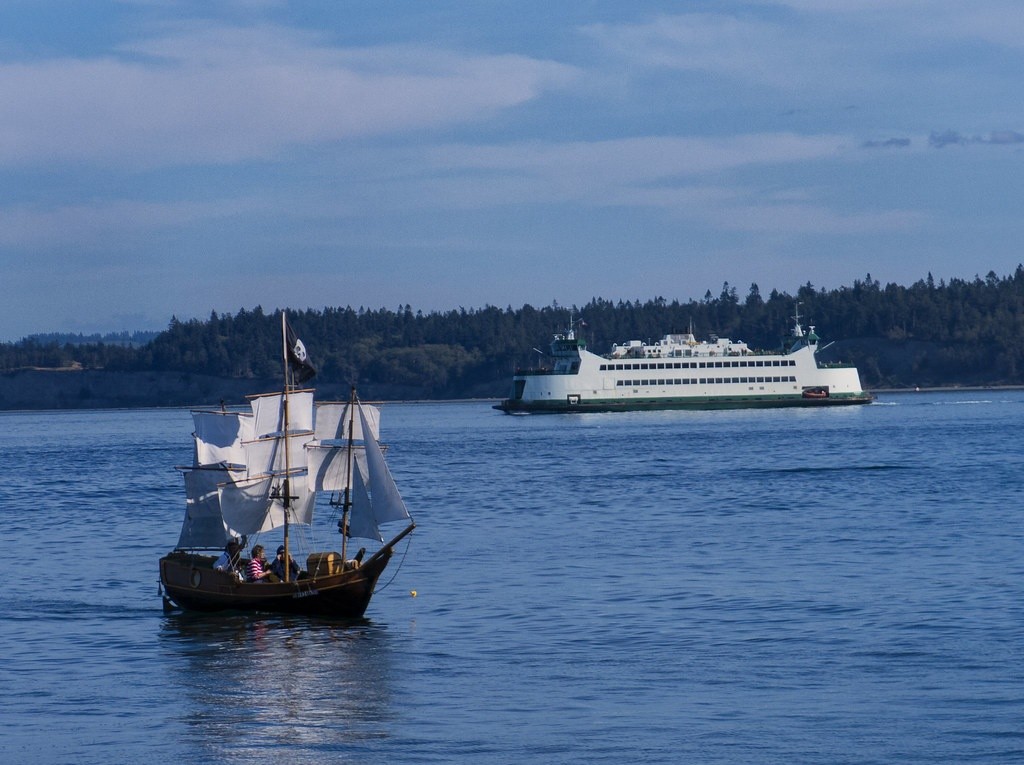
801;389;827;397
492;306;875;412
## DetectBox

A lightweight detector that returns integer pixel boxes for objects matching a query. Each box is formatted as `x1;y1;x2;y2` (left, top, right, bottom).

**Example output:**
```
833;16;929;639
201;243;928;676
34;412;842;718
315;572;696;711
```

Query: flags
285;320;317;386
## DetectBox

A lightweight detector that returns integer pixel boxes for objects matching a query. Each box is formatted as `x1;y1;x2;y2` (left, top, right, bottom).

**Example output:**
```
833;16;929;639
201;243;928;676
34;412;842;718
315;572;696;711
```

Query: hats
277;545;285;555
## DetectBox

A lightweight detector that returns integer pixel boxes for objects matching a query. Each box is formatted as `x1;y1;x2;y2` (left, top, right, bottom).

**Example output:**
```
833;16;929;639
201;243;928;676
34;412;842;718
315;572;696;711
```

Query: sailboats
156;311;417;624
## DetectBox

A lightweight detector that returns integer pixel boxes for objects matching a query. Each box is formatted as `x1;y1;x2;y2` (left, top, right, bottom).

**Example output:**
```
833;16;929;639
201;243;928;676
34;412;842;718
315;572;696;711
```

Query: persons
212;541;248;582
271;545;300;582
246;544;272;583
